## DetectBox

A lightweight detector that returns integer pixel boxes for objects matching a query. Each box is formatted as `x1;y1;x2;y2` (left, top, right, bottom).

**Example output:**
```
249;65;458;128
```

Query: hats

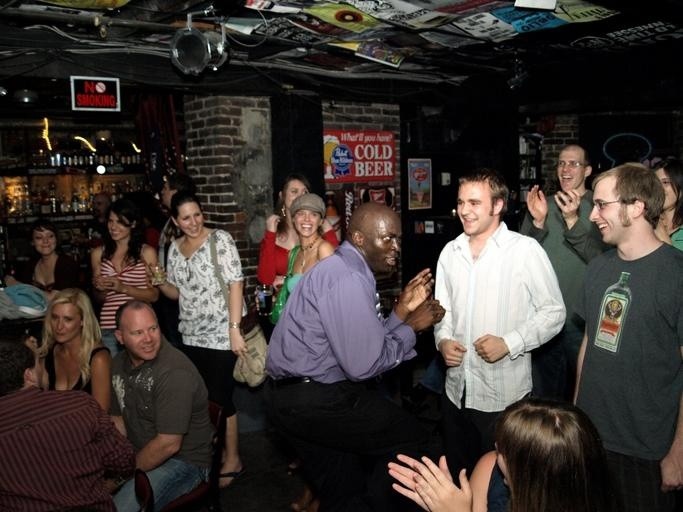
291;193;326;219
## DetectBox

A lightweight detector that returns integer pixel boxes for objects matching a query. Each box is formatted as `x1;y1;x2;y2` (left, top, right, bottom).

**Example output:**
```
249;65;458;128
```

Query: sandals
286;459;307;478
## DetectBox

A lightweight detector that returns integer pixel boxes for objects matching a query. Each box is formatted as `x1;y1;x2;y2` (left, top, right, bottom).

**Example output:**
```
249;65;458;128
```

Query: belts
269;376;309;388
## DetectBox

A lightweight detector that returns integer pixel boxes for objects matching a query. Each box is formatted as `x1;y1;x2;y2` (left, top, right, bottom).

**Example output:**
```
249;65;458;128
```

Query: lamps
171;5;229;76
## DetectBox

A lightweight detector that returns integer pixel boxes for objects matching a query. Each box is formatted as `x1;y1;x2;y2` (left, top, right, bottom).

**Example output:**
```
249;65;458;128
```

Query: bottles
105;180;152;202
592;271;633;355
5;180;57;215
57;182;104;215
32;149;142;167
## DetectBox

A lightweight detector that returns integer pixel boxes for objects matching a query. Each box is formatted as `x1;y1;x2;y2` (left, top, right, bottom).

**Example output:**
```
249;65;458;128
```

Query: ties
376;293;381;322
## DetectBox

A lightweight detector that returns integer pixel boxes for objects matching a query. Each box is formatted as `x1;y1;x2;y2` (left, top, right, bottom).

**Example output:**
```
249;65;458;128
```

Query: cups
255;283;273;316
149;263;164;286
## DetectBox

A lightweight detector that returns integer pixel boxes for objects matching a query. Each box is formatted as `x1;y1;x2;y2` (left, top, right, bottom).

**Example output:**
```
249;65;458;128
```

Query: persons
256;171;339;291
519;142;611;405
430;167;568;472
257;199;463;510
270;192;336;298
158;171;197;272
650;156;683;252
568;161;683;512
90;196;160;335
386;396;625;512
1;214;220;512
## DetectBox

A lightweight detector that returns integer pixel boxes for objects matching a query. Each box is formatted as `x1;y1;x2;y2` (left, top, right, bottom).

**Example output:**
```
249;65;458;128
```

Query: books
353;38;406;68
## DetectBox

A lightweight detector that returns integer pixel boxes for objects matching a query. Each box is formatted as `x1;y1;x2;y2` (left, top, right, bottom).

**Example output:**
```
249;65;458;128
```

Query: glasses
556;161;586;168
591;200;620;209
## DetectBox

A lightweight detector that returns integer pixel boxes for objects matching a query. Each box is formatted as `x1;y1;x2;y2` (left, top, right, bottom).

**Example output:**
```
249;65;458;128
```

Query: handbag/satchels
232;322;267;388
0;284;48;320
272;245;301;325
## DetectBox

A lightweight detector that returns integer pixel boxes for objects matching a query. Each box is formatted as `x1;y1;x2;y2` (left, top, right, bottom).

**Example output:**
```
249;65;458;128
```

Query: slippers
216;463;246;489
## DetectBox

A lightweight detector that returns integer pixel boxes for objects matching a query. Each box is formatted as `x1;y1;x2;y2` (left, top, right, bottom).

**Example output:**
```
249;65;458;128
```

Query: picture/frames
407;158;432;210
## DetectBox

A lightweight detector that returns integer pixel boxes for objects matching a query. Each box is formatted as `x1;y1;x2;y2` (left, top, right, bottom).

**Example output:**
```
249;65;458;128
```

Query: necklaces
300;233;321;252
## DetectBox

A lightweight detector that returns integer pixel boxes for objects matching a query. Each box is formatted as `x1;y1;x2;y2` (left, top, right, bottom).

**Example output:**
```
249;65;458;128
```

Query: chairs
135;469;154;512
162;400;223;512
